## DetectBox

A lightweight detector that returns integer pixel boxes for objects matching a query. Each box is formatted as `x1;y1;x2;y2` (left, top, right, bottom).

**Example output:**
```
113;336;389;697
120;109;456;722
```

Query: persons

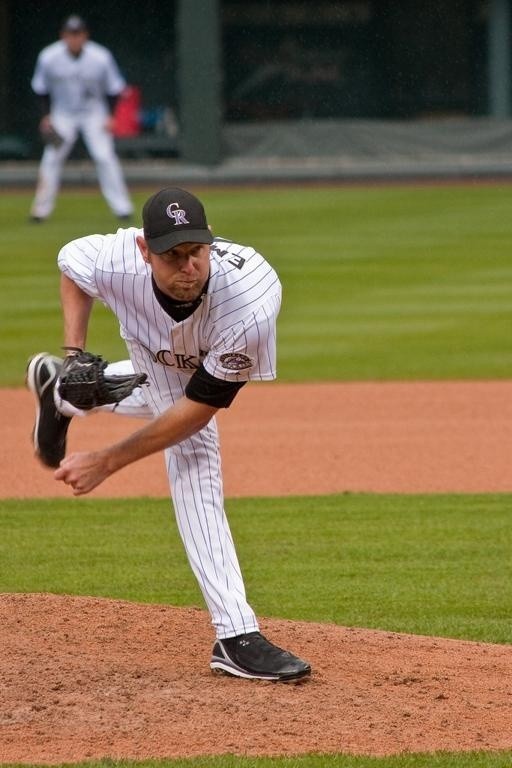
20;181;314;683
20;9;144;230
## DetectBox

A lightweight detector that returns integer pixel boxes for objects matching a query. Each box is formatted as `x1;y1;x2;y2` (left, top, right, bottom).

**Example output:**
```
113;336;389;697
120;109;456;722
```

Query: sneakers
25;351;73;470
210;633;311;684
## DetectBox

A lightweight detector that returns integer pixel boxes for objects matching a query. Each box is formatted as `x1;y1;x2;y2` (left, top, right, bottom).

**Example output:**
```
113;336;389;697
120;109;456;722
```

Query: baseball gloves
59;348;147;412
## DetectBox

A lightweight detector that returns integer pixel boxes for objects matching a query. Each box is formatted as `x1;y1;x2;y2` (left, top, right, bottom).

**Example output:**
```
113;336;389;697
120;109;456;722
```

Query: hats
64;15;85;33
142;186;213;255
